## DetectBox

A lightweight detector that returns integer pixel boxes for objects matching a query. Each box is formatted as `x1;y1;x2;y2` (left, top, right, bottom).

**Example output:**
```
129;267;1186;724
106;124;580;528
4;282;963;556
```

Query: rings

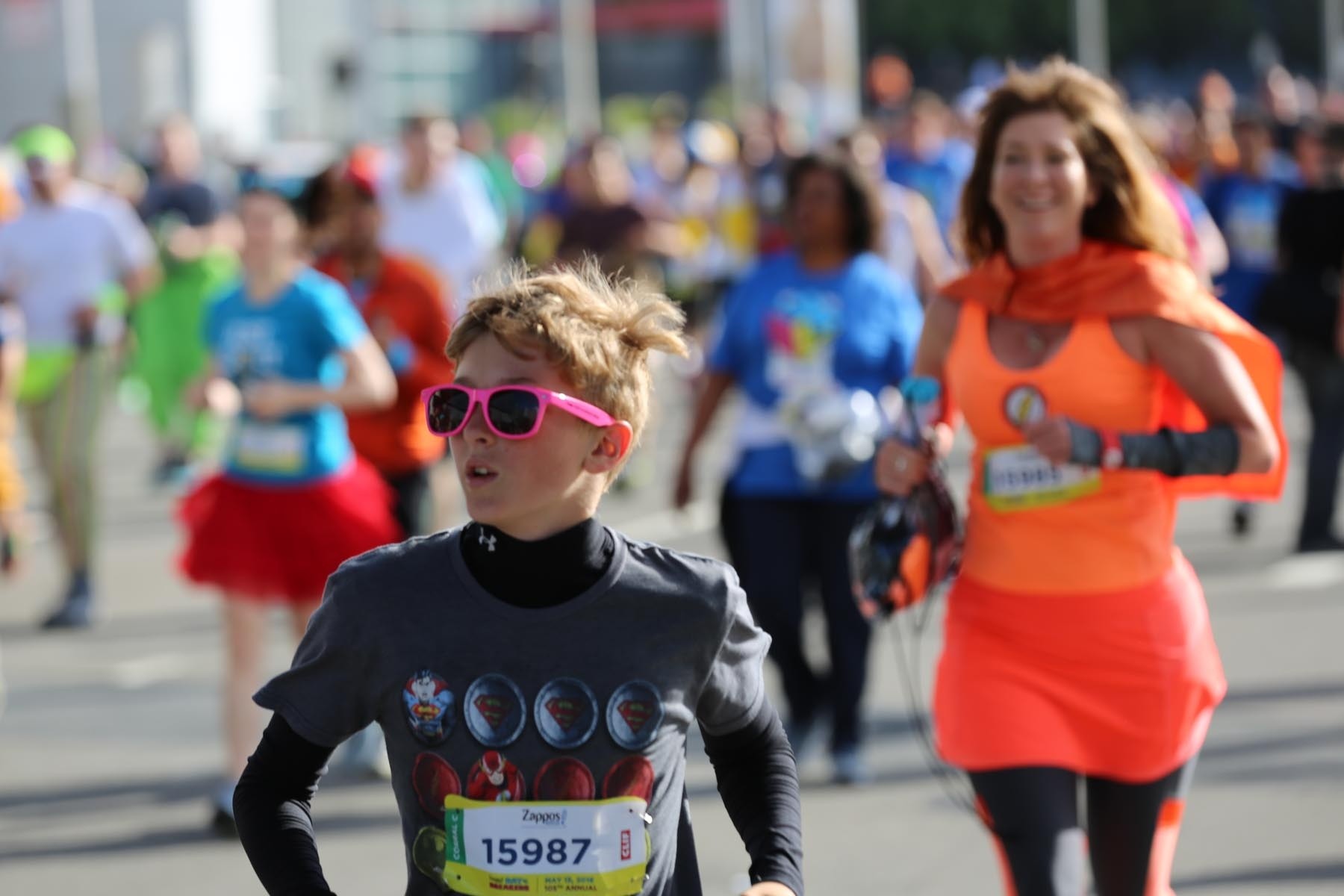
897;458;905;473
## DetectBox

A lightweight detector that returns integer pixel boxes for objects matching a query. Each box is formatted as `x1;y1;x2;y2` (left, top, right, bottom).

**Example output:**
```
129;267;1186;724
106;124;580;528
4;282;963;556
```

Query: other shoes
218;776;250;830
827;734;865;788
778;716;827;767
46;577;100;627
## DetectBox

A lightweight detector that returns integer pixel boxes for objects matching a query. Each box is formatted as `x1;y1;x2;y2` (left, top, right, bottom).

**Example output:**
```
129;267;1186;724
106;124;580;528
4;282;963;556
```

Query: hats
16;122;76;165
329;146;391;197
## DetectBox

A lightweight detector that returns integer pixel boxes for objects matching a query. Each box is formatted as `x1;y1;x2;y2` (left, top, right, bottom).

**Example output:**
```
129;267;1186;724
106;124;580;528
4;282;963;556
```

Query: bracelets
1097;428;1123;468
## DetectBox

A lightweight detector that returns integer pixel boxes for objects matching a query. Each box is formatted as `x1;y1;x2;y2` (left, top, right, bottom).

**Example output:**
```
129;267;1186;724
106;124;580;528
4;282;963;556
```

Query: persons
667;148;934;784
232;251;803;895
875;56;1289;896
175;185;397;828
0;68;1344;629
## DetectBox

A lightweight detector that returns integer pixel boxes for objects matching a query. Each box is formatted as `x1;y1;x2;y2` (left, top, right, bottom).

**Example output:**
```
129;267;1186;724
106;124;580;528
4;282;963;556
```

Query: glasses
420;383;617;440
